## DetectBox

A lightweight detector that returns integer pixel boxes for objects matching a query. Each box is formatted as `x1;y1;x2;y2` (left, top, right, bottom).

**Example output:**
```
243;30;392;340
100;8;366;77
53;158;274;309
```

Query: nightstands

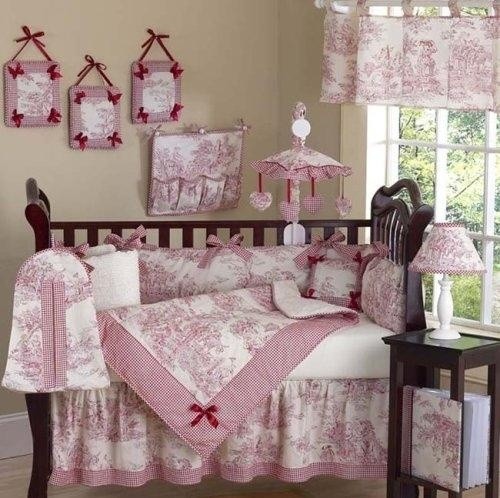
384;327;499;497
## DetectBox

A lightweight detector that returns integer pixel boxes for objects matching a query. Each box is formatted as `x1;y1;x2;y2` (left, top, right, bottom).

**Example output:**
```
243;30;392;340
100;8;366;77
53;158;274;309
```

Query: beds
22;175;435;497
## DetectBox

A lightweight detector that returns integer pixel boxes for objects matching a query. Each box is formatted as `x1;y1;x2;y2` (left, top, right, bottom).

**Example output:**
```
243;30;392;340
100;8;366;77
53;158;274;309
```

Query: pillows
309;256;406;336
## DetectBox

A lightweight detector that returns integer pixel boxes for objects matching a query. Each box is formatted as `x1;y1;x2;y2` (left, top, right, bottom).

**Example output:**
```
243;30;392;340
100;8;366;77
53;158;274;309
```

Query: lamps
409;222;488;341
251;102;353;223
